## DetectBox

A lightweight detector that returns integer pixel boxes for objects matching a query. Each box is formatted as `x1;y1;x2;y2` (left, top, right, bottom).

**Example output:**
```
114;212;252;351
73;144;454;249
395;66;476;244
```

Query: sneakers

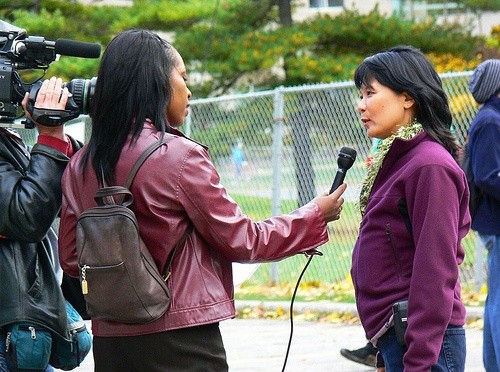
340;342;378;367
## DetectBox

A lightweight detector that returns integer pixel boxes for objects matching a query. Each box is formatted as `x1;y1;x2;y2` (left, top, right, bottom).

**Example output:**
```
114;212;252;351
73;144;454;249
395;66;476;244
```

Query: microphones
330;146;357;196
25;36;101;58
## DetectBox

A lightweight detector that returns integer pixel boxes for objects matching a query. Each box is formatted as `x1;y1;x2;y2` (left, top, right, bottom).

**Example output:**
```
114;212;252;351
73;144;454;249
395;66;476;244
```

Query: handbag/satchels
5;296;92;372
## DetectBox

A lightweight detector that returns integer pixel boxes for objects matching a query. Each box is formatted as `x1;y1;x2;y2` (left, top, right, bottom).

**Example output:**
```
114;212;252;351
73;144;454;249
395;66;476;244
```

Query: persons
459;58;500;372
0;29;472;372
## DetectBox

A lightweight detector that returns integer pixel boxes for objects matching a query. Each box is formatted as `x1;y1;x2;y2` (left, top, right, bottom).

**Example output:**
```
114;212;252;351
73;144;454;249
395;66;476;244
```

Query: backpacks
76;135;194;323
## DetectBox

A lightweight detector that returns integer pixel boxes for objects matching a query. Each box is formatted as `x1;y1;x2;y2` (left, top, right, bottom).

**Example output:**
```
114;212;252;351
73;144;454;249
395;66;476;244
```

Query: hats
468;59;500;103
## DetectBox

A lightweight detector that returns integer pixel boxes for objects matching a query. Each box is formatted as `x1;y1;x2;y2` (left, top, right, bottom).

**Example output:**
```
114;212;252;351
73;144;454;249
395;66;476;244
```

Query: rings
338;206;343;212
